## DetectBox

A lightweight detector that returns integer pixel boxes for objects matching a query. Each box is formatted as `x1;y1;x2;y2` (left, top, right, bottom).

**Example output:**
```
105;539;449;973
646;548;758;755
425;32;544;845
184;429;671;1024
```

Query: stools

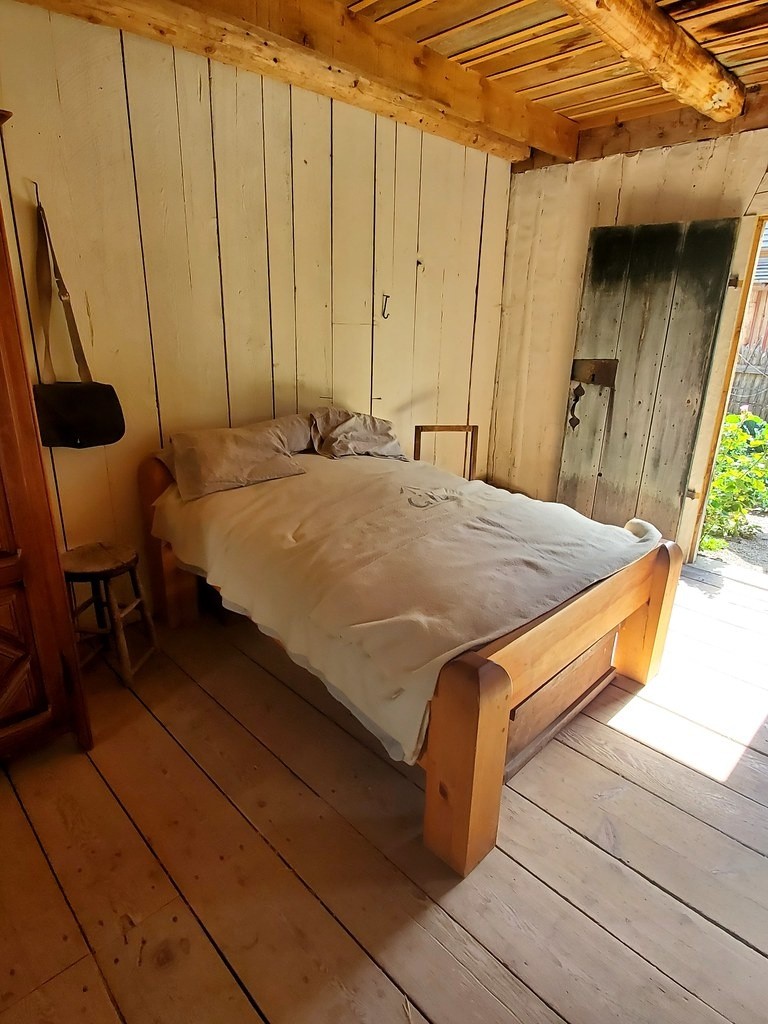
58;540;161;690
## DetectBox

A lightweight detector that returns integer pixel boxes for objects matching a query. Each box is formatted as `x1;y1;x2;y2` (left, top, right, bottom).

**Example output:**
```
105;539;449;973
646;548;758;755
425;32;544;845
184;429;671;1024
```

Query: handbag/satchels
31;380;129;446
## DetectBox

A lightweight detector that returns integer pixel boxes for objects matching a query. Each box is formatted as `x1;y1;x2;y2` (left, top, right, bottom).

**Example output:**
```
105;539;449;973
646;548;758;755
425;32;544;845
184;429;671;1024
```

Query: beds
135;421;683;879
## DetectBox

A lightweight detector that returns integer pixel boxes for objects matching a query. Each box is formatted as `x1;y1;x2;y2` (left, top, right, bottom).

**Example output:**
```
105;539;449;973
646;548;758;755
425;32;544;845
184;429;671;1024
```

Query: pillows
166;423;308;503
311;407;410;463
